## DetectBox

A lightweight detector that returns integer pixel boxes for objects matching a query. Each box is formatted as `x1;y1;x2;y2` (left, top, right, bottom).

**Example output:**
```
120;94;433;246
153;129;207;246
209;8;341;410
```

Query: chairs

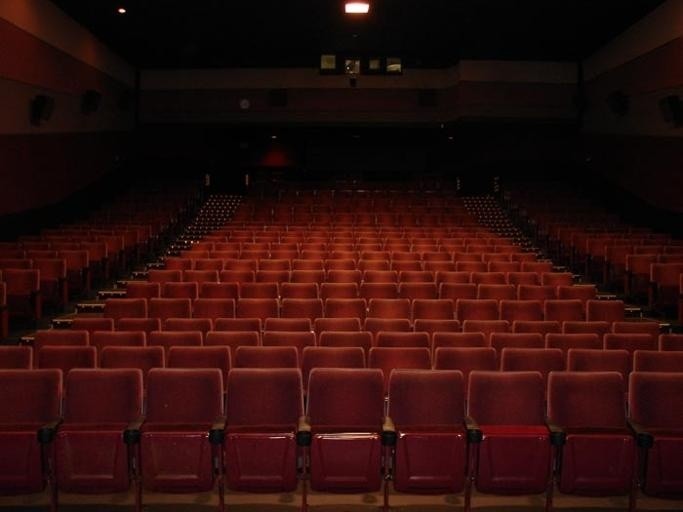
1;173;683;512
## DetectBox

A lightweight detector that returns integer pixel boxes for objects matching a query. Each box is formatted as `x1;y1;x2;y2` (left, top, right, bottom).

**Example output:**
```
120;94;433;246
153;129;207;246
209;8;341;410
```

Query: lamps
656;95;683;129
319;50;403;80
80;88;102;114
31;95;57;125
